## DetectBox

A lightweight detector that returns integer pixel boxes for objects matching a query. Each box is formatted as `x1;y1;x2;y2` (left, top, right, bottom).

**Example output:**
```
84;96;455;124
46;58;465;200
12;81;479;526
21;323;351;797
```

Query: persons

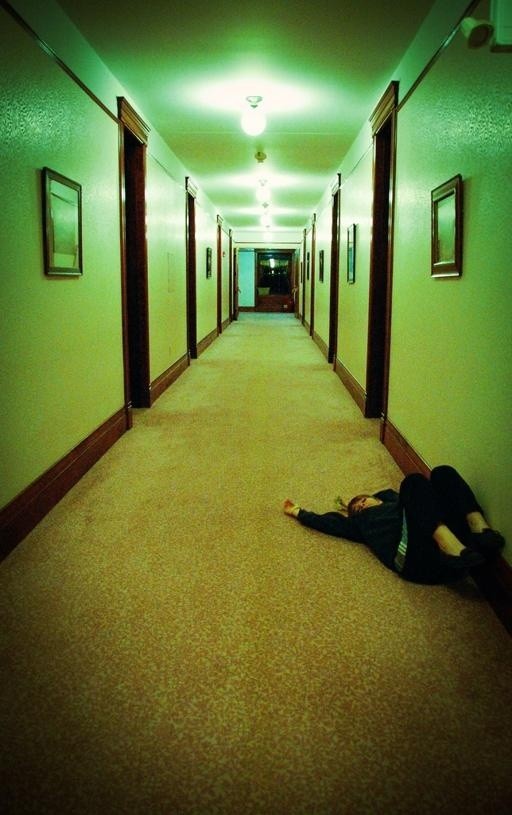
282;464;507;595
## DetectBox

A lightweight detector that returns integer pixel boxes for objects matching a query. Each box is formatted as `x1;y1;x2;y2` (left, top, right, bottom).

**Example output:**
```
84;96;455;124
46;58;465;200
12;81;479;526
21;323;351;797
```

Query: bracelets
290;505;301;516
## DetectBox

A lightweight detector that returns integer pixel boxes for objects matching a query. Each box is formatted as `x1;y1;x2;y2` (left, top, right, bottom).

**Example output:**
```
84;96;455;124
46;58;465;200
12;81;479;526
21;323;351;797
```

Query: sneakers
436;528;506;566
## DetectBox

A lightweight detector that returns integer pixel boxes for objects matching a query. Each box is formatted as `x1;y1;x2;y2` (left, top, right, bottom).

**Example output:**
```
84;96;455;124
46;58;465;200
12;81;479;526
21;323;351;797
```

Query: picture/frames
341;222;359;288
420;172;471;287
34;158;91;284
